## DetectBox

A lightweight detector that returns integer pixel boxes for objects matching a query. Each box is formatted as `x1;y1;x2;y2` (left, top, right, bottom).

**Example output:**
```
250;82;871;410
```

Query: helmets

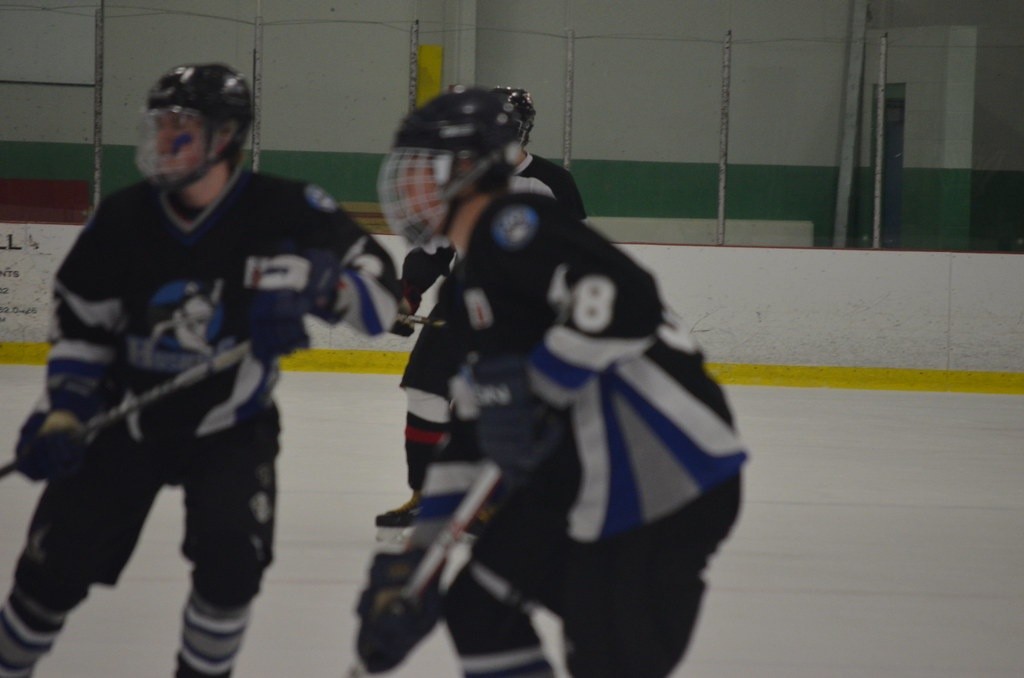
492;85;537;147
135;63;255;187
376;84;525;248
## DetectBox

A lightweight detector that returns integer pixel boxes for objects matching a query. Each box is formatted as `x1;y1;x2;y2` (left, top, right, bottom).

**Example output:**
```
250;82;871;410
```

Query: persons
0;62;398;678
355;86;747;678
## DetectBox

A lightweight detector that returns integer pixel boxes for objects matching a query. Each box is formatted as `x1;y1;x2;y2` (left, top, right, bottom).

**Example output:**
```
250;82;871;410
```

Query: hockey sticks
346;465;504;678
0;339;252;483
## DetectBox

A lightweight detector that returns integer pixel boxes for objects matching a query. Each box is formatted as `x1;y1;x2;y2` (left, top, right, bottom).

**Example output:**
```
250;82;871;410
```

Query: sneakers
373;493;423;544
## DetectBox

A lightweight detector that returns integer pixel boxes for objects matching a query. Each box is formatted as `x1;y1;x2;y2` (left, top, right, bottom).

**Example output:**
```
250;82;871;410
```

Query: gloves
247;254;310;355
473;390;576;477
15;411;87;482
390;279;424;337
356;553;450;674
